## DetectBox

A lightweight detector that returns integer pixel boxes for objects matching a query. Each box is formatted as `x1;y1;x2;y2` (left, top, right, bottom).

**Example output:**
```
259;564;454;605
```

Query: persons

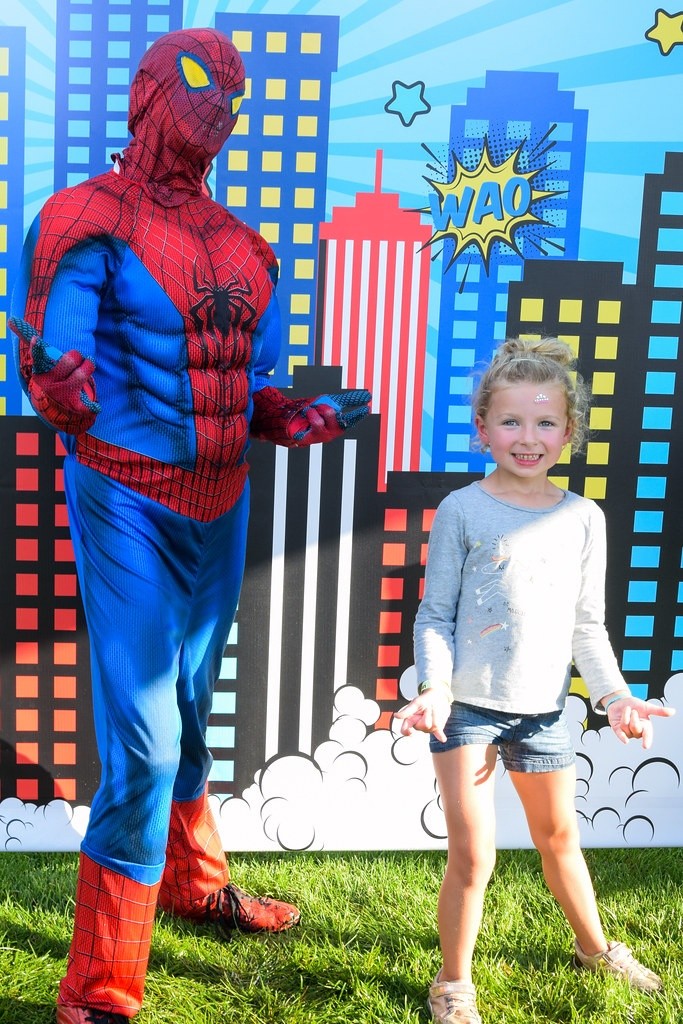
390;336;677;1024
5;27;372;1024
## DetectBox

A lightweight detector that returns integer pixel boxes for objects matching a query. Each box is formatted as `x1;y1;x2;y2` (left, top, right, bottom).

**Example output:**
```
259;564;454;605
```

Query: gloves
8;316;102;415
287;390;372;446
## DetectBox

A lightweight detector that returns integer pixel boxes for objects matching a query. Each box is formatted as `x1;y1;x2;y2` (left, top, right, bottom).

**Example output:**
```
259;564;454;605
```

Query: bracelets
605;693;628;714
420;679;454;703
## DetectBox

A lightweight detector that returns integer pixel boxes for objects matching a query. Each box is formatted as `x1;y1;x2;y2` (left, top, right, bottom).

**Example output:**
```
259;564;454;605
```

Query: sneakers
57;1004;128;1024
189;882;303;941
427;966;481;1024
574;937;663;990
